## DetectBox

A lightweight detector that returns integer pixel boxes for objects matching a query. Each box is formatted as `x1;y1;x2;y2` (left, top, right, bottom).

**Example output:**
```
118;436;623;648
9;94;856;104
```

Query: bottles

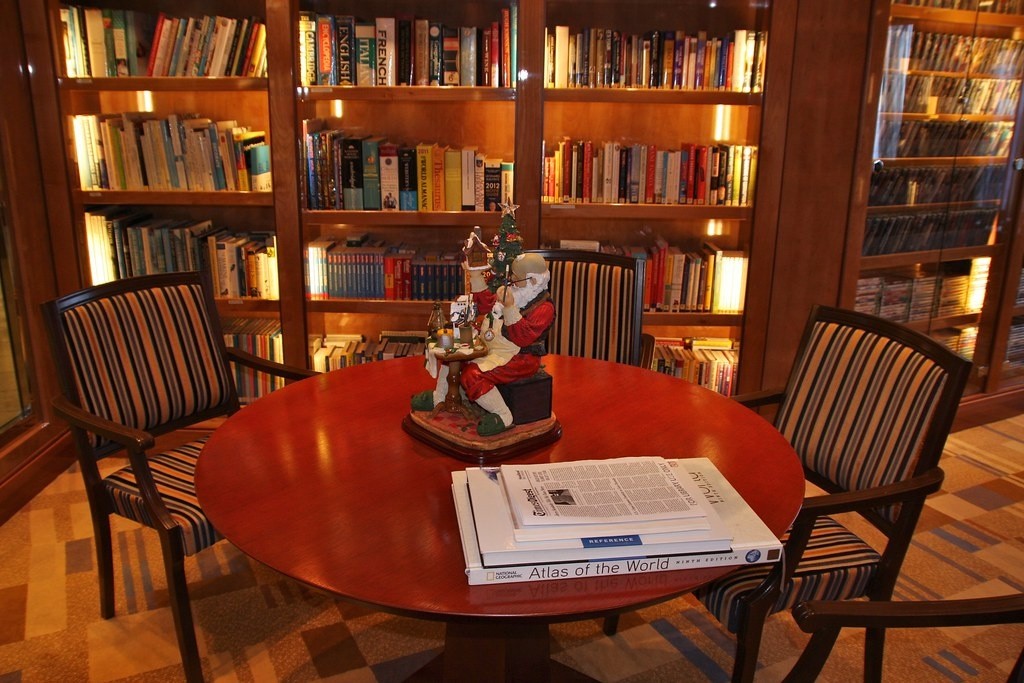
428;302;446;339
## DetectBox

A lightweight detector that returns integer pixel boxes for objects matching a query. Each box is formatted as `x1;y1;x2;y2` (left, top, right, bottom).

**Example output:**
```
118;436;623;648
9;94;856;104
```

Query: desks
194;354;806;683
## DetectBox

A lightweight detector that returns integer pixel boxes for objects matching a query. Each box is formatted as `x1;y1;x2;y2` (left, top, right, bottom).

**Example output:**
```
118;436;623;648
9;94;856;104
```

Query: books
1015;270;1024;305
306;330;428;372
545;26;767;92
540;240;749;314
854;257;991;323
890;0;1022;14
84;212;276;298
920;325;979;364
867;164;1008;206
652;335;741;398
542;136;760;206
219;315;285;397
60;6;267;76
883;32;1024;75
449;456;784;587
874;120;1015;156
860;208;998;257
303;234;496;302
298;119;515;212
299;3;516;87
878;72;1022;114
1005;326;1024;362
73;114;272;191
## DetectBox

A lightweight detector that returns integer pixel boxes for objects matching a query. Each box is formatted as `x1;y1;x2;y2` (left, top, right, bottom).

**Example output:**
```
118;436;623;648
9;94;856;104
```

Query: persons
410;253;557;436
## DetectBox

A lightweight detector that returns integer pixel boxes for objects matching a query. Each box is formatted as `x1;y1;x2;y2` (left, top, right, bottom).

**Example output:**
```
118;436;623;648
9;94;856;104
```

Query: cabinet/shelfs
834;0;1024;437
19;0;837;461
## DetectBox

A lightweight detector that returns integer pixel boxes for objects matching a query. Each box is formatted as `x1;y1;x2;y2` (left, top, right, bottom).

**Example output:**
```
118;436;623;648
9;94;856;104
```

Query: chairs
40;270;324;683
524;247;648;369
600;301;975;683
